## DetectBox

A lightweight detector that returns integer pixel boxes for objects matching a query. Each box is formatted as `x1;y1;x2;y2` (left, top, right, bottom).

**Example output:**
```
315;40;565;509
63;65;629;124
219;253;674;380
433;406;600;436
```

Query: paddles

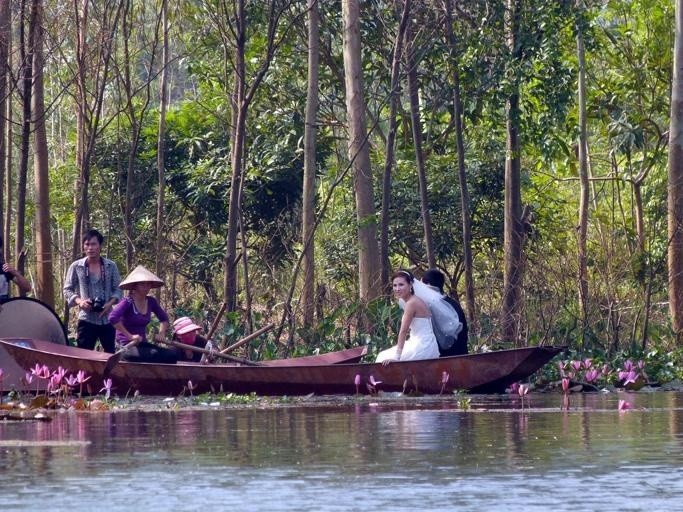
104;340;138;375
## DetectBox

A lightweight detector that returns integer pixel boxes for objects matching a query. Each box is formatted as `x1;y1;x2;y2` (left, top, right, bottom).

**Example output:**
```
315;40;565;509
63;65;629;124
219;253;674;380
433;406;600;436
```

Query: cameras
90;297;105;312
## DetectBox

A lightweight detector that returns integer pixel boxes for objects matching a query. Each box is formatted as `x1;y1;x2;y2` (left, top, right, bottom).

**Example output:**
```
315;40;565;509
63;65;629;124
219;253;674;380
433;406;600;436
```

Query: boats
0;324;568;394
176;341;368;366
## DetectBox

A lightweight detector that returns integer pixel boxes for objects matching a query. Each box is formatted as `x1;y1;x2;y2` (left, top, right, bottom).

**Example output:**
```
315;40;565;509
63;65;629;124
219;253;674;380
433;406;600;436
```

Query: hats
117;265;165;290
172;316;203;335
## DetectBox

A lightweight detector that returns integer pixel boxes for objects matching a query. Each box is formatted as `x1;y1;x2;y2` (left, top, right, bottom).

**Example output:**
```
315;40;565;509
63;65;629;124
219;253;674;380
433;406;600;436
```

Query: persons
378;270;440;368
0;255;30;302
167;316;219;365
108;264;178;363
418;269;467;355
61;230;124;355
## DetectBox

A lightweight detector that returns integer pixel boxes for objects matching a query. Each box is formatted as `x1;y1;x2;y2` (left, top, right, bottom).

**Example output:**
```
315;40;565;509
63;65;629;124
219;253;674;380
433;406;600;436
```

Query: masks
176;335;196;345
